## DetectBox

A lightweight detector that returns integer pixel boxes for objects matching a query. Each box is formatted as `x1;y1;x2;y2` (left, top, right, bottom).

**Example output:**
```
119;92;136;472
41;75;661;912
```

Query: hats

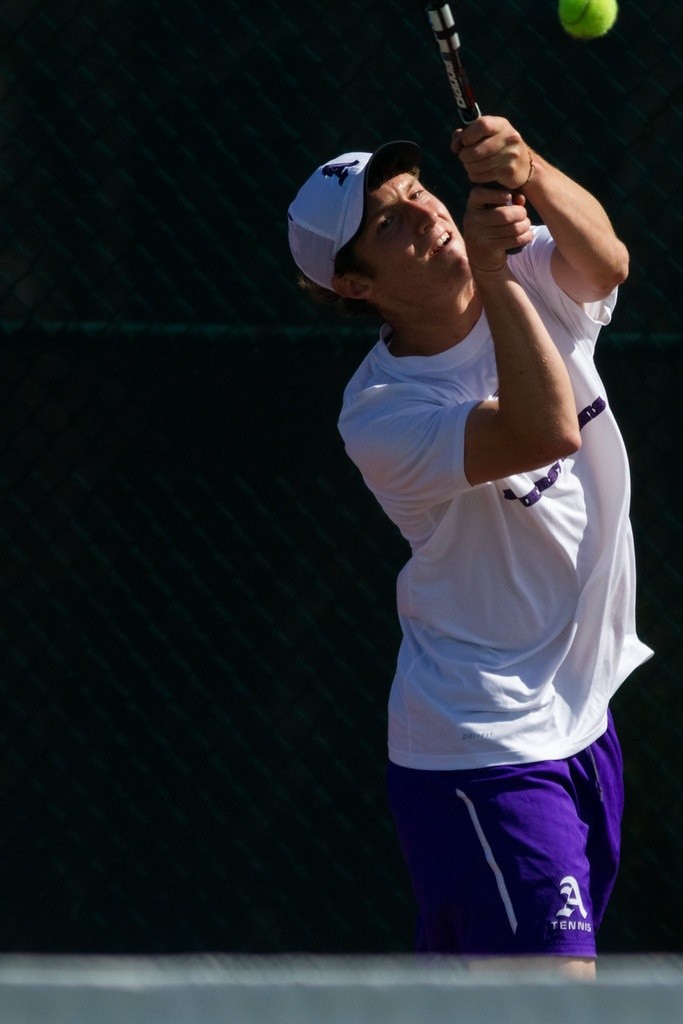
287;140;423;294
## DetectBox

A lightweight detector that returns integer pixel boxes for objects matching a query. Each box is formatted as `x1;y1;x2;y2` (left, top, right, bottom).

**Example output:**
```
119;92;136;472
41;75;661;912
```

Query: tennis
556;0;620;39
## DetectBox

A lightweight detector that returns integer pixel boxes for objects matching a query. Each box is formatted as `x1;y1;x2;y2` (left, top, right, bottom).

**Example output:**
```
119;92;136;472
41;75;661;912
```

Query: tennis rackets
423;0;526;256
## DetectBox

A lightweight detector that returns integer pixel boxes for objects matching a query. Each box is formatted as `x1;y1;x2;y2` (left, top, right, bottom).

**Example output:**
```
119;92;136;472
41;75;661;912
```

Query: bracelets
515;147;534;189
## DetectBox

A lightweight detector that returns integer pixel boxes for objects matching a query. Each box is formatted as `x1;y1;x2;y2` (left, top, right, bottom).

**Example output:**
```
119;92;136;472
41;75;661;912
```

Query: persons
288;115;654;979
